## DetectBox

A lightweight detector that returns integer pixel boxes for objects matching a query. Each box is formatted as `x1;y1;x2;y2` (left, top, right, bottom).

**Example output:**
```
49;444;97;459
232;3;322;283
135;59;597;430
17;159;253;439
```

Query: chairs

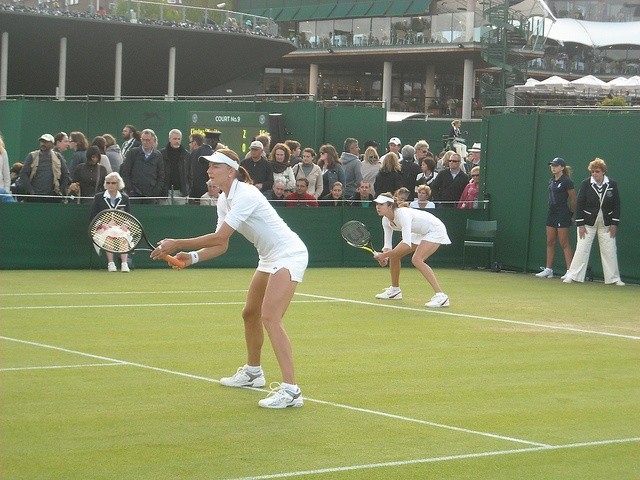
462;218;497;269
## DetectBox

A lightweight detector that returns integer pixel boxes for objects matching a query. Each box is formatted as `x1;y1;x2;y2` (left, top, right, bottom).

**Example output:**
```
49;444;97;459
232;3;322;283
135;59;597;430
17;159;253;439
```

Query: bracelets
189;250;199;265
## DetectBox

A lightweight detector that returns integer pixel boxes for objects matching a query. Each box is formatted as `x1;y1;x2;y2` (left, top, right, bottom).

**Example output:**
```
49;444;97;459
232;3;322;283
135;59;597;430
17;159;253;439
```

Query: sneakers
563;277;574;284
121;262;129;271
613;279;625;287
375;288;402;300
107;262;117;272
561;272;568;280
535;268;553;279
219;367;266;389
257;382;304;410
424;293;450;308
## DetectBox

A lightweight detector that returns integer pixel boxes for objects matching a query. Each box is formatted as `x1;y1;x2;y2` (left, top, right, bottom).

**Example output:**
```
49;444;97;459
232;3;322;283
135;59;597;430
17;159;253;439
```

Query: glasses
189;141;195;143
140;137;152;142
471;173;480;177
448;160;461;163
420;150;428;153
591;169;602;173
69;139;76;142
369;154;377;158
106;181;117;184
39;140;49;144
321;151;327;155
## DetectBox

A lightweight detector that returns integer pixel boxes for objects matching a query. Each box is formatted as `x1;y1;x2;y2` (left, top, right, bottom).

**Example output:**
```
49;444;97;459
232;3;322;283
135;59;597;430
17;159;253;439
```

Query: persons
204;131;227;153
414;139;430;165
0;137;11;194
399;145;420;200
200;179;221;205
263;180;284;206
535;157;576;279
120;129;164;204
149;148;310;410
374;152;409;199
292;147;324;199
456;166;480;209
361;146;383;197
411;185;436;208
430;154;469;201
73;145;107;204
319;182;348;206
244;134;270;160
158;128;194;205
90;135;113;174
317;144;345;206
564;158;625;286
53;132;69;155
268;143;295;186
393;187;411;208
380;137;404;168
17;134;72;204
121;124;142;159
69;132;89;180
339;137;362;200
415;157;438;201
284;178;318;207
103;133;123;173
188;134;213;204
90;172;131;273
238;141;274;191
372;192;452;309
285;140;303;167
348;181;375;207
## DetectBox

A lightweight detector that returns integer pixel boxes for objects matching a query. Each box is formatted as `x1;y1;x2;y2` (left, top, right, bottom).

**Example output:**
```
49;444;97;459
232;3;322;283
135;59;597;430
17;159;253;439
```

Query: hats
387;136;401;146
37;134;54;144
549;157;567;167
467;143;482;152
203;132;222;141
197;151;240;170
366;139;380;147
249;140;263;150
373;195;395;205
